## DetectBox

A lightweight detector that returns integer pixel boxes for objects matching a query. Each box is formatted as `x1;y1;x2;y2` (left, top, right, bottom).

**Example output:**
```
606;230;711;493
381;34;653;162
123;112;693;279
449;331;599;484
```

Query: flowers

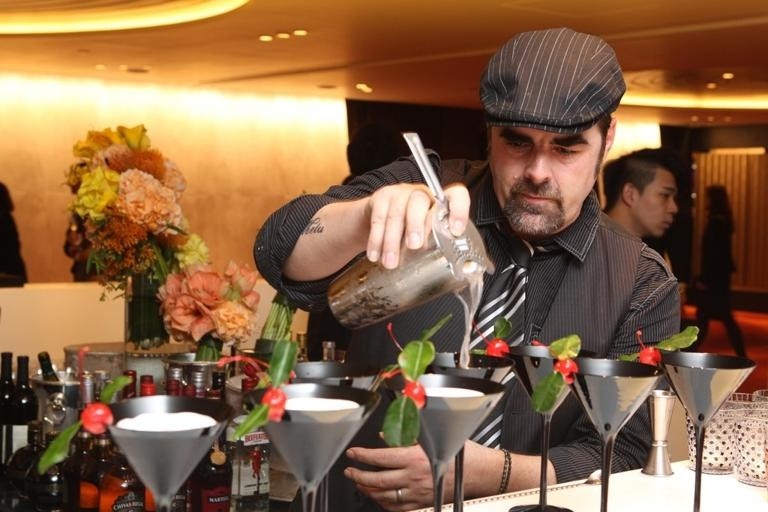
147;255;283;368
57;122;214;303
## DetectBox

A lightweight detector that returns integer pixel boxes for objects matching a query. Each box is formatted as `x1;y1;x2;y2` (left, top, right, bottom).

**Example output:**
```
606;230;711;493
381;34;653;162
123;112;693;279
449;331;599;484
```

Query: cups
645;391;676;476
326;199;486;330
685;388;768;488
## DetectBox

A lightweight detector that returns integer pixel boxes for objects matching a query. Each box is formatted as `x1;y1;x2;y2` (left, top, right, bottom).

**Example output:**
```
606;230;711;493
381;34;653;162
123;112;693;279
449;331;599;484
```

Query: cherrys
639;347;663;367
487;339;510;357
80;402;114;435
404;382;426;409
261;388;287;423
553;359;578;384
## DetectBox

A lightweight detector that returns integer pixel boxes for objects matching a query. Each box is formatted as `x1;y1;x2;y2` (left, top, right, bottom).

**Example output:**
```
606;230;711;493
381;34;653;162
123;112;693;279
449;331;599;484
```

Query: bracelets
499;445;513;497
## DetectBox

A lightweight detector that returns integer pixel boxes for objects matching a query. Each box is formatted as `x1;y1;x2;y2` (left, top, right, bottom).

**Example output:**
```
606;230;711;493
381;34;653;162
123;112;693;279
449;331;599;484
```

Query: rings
397;488;404;504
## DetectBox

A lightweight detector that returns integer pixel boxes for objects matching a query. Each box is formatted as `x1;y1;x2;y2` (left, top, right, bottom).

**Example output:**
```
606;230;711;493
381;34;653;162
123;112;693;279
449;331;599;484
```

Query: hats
479;28;626;135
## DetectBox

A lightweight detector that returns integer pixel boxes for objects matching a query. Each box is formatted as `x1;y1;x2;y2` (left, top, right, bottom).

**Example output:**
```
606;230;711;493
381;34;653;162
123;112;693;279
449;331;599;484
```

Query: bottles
2;350;269;512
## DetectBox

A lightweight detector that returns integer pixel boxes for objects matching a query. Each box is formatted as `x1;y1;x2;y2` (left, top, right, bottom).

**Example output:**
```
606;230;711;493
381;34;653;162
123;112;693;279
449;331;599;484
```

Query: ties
467;222;563;449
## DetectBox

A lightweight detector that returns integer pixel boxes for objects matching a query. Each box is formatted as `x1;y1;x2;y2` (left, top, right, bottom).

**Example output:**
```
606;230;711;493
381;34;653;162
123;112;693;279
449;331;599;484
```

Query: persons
693;183;750;362
601;147;683;254
251;25;679;510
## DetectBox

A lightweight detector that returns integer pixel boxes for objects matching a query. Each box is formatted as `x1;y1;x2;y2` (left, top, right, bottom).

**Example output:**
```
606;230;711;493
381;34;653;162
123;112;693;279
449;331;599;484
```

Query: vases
118;267;170;352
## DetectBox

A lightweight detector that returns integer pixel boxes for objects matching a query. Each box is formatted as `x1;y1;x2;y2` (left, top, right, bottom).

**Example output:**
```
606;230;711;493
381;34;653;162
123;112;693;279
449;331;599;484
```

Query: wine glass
659;348;755;511
263;381;380;509
283;356;386;510
425;348;512;512
390;372;504;512
504;342;596;512
566;354;658;511
